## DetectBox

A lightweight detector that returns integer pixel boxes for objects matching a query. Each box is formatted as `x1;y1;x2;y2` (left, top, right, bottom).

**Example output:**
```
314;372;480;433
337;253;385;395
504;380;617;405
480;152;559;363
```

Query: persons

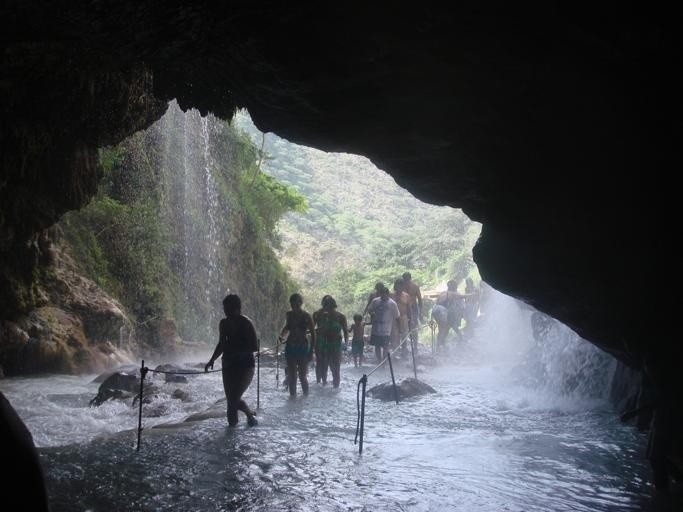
204;293;261;429
277;270;489;396
